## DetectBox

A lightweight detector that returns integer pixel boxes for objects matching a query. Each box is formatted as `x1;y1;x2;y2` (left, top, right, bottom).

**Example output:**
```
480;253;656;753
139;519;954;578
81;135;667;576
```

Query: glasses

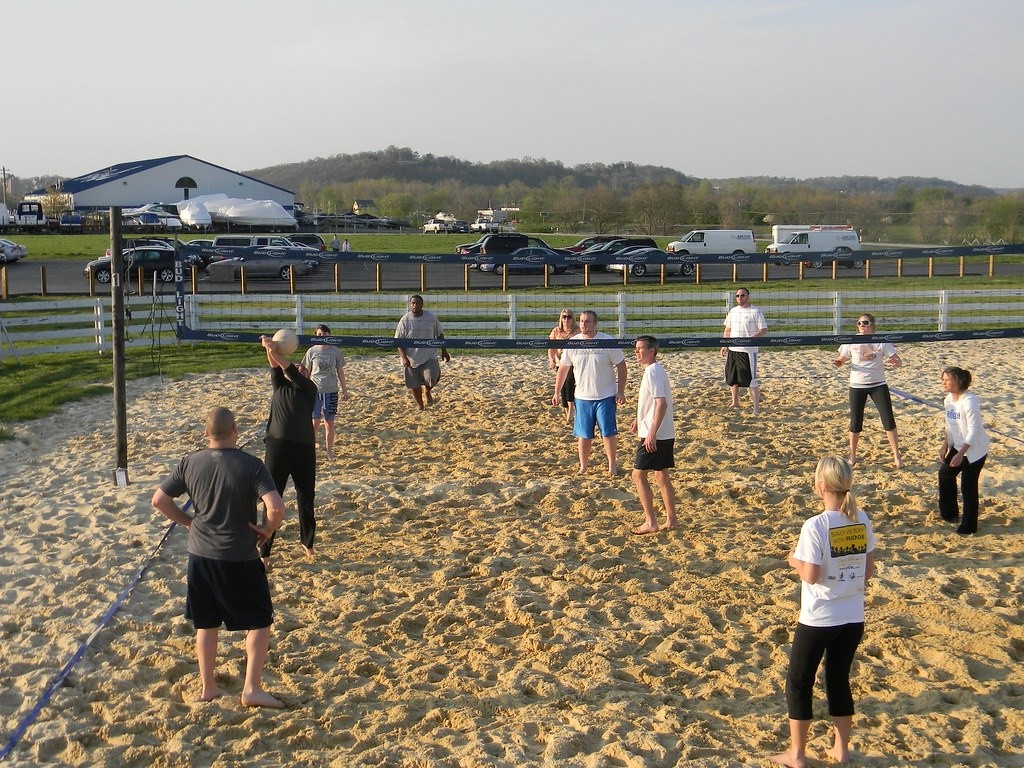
857;321;873;327
562;315;573;320
735;294;747;298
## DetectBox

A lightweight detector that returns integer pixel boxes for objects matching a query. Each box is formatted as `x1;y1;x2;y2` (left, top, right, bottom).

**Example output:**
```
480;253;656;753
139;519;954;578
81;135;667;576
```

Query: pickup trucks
423;219;453;234
470;218;499;234
14;200;46;232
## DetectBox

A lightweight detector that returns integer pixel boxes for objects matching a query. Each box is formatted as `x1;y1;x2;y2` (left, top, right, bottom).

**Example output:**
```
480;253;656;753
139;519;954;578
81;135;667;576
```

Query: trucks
666;229;757;255
772;225;854;244
764;230;866;270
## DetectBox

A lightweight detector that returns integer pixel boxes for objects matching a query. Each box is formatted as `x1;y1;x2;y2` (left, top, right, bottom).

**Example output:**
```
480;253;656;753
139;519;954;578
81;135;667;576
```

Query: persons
552;311;627;477
766;455;877;768
629;335;679;533
548;309;581;424
343;239;349;252
932;365;989;534
301;323;350;462
258;335;318;559
835;314;905;469
394;295;450;412
720;287;768;415
152;408;285;707
330;234;342;252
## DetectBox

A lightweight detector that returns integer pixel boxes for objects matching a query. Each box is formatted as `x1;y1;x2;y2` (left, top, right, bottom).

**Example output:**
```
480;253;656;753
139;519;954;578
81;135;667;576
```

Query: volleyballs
272;329;298;355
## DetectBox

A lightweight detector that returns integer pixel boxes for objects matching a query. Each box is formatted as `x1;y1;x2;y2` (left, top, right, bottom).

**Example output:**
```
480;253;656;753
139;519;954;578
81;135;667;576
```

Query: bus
97;210;161;225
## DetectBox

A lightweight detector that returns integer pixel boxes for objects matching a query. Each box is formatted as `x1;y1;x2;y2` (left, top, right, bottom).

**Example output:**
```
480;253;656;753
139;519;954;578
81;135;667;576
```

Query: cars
456;232;699;276
59;209;83;235
82;233;327;285
0;238;29;265
444;219;471;234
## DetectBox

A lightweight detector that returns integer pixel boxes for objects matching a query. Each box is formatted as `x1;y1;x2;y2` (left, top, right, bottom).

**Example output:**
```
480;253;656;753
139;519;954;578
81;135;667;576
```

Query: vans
0;203;10;233
